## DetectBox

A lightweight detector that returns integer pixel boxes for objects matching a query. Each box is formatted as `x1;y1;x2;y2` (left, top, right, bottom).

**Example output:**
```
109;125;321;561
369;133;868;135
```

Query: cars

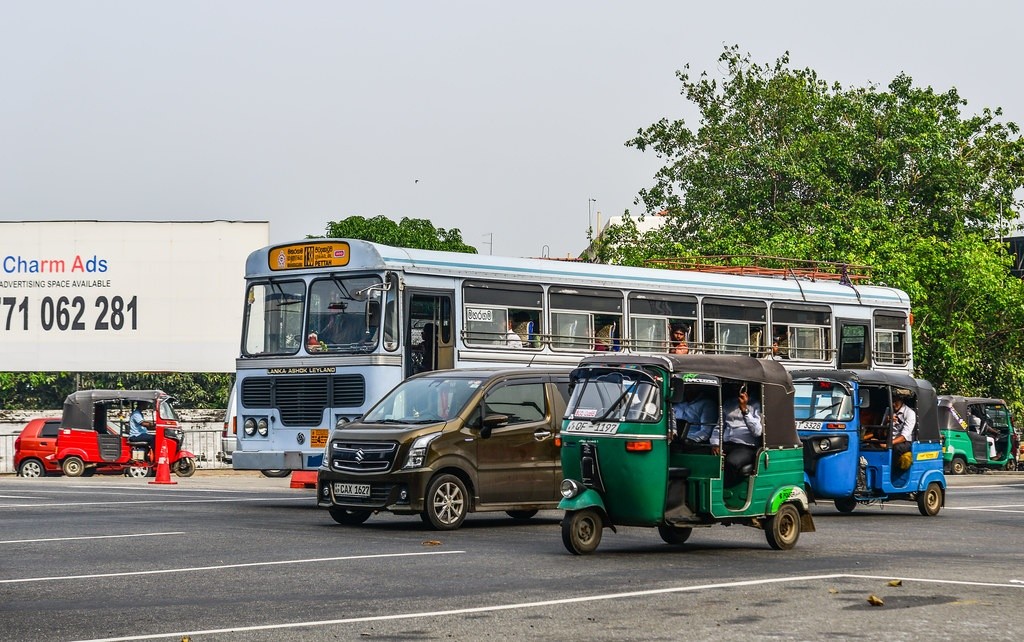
14;418;156;478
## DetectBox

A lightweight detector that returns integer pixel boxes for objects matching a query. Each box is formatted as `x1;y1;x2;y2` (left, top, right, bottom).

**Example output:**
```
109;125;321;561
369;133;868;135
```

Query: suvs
317;367;622;531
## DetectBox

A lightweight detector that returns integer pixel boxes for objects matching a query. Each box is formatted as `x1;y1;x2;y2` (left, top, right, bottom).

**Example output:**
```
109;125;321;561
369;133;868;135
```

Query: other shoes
992;452;1001;461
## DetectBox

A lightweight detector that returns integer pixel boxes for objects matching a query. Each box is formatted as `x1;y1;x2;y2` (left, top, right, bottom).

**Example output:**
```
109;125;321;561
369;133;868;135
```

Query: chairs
511;321;827;362
125;425;149;446
669;419;691;477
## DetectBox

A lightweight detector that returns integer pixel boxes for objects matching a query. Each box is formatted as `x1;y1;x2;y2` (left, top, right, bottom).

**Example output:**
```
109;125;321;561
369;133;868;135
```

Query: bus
233;238;914;471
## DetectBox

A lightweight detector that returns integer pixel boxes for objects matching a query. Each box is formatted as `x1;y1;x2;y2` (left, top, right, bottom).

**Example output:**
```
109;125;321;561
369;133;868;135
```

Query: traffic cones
148;438;177;484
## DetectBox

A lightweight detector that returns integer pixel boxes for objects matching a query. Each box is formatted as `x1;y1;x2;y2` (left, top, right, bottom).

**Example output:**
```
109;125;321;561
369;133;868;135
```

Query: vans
216;378;293;478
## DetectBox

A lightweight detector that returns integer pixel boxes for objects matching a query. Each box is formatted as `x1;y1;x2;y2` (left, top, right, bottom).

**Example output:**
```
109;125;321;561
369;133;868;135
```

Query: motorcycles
790;370;947;517
55;389;197;477
555;354;815;554
937;395;1024;476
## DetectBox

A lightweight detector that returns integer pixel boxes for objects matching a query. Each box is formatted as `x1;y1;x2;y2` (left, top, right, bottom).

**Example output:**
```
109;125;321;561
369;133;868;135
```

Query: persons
129;401;154;450
491;316;524;349
710;384;763;486
662;322;689;354
416;323;438;372
865;395;917;470
773;338;782;360
671;384;714;446
969;408;1002;460
586;325;608;351
